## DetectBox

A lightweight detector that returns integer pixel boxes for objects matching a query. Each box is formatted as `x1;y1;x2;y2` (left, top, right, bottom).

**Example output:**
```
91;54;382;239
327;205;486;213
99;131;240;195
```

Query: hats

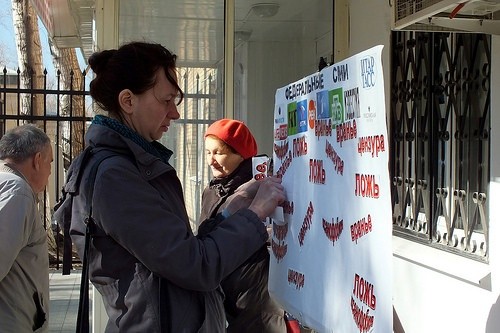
205;119;257;159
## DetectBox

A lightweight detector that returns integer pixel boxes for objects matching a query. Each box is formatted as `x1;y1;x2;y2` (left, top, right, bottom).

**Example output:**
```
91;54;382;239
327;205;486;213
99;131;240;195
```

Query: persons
0;124;53;333
55;42;287;333
197;119;287;333
